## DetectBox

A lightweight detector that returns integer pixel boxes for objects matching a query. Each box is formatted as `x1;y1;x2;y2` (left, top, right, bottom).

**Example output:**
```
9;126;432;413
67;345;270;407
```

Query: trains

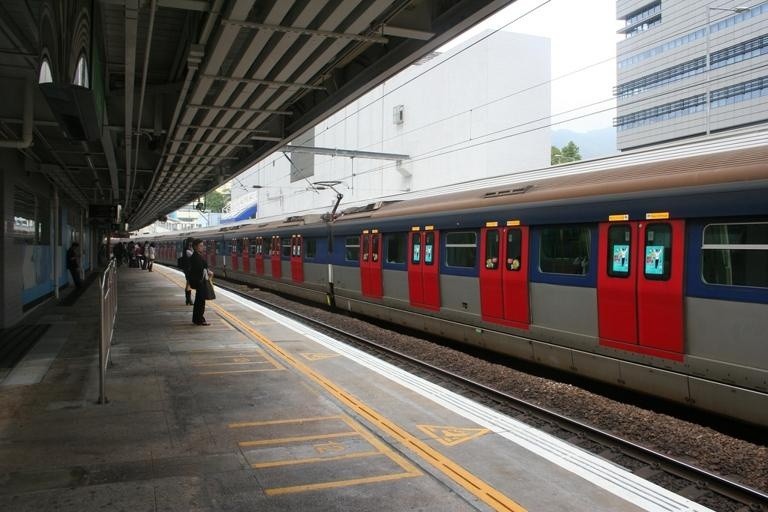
110;145;767;426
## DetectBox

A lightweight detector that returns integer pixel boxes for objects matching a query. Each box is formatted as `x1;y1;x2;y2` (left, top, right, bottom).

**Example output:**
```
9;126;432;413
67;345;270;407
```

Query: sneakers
193;319;211;327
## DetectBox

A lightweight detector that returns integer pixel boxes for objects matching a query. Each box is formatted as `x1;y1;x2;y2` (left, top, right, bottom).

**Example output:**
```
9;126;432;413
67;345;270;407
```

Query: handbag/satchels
203;270;216;301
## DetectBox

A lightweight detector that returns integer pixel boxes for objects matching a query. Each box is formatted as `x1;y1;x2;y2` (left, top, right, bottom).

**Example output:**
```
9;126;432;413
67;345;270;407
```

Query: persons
616;245;662;270
180;236;195;306
113;241;157;272
188;238;215;327
65;241;83;290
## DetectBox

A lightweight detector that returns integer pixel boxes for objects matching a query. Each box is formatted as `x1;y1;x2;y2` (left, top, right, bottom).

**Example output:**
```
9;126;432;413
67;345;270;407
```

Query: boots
183;287;196;307
